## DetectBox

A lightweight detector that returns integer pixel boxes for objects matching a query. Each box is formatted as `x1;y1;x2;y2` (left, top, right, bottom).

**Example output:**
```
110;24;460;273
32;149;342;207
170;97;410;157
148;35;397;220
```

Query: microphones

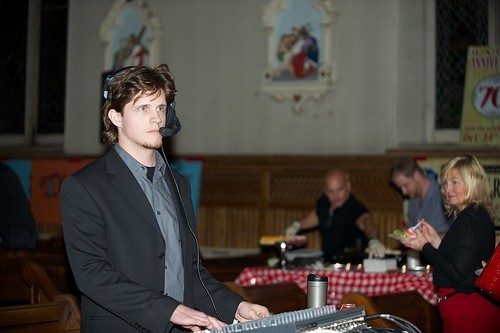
160;117;182;136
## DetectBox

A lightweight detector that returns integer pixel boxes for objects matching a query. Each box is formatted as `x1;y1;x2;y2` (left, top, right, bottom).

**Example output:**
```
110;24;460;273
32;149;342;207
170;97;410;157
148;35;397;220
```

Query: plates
388;234;416;241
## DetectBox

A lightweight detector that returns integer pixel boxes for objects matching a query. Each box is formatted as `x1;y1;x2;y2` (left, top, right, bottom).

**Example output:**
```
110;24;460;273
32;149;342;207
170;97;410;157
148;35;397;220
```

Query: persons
401;154;500;333
391;157;454;240
286;168;385;267
61;64;271;333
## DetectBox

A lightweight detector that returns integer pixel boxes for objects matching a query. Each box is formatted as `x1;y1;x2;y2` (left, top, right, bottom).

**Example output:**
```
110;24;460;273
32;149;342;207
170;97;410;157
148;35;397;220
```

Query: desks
0;244;267;296
236;267;438;333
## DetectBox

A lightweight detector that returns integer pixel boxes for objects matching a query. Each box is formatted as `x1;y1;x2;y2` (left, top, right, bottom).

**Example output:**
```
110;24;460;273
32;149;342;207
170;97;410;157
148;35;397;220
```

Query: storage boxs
364;258;397;273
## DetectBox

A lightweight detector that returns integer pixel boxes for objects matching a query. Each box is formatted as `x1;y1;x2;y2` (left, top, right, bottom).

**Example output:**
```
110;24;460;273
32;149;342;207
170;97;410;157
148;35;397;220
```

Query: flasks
307;274;328;309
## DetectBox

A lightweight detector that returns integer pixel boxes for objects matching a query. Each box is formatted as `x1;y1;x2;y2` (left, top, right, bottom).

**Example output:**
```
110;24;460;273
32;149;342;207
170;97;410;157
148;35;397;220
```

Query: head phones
101;67;177;127
